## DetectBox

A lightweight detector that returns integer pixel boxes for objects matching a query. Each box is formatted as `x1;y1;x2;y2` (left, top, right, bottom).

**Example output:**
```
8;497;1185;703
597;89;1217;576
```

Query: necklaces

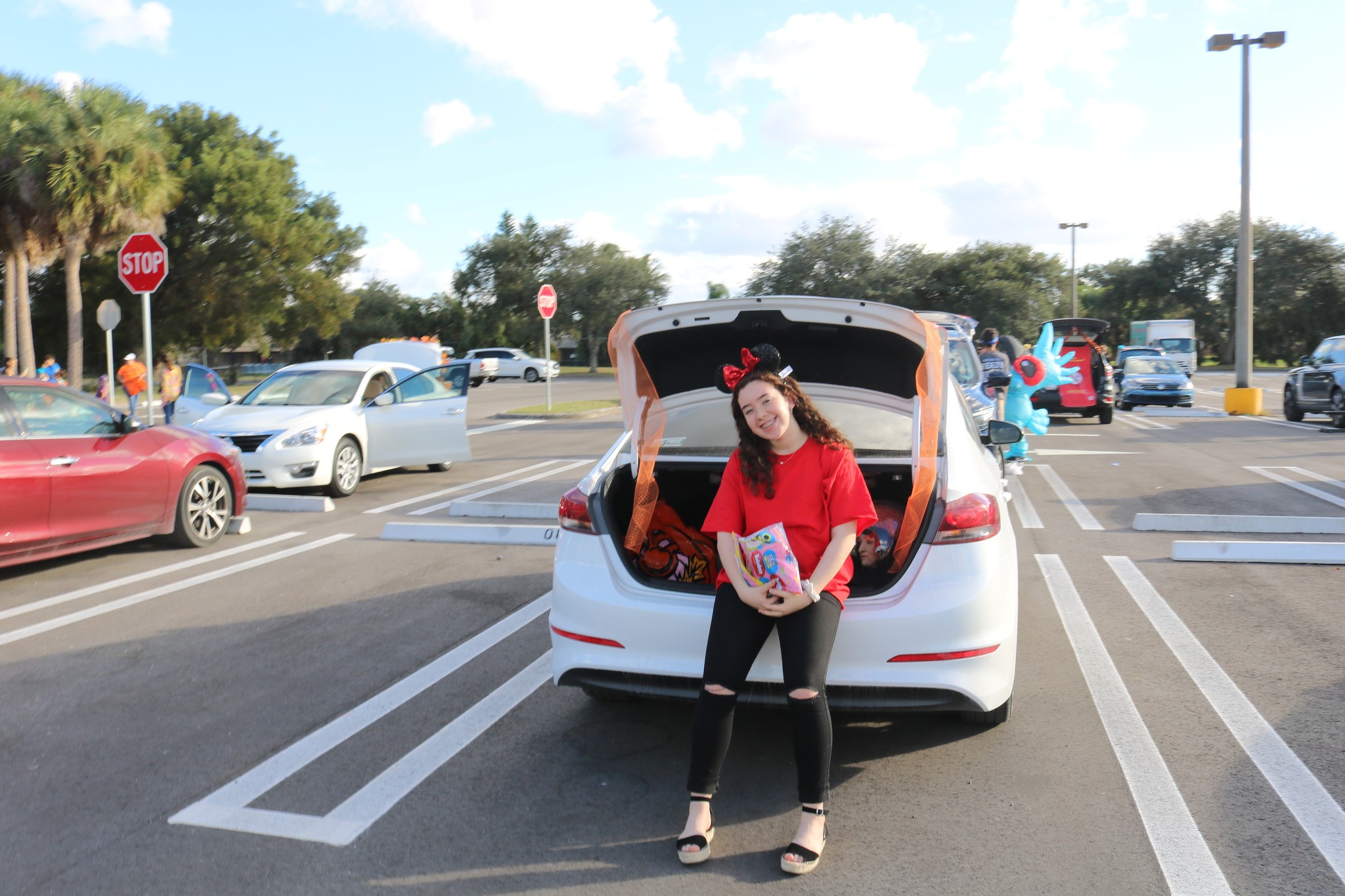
772;432;804;465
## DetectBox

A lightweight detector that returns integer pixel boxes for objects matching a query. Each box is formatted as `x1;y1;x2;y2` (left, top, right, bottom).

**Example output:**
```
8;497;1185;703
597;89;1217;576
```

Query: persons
96;374;110;403
677;343;878;875
154;348;183;424
0;353;68;387
116;353;147;418
852;503;903;577
974;319;1026;462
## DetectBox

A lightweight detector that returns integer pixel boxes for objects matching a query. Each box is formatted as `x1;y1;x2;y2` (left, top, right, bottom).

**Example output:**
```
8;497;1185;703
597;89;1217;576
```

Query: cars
0;377;247;569
548;295;1018;726
1112;356;1195;411
441;348;560;386
170;340;471;497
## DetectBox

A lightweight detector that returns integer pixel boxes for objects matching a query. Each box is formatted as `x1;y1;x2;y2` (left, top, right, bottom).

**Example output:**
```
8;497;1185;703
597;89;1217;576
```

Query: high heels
676;794;715;865
781;803;828;874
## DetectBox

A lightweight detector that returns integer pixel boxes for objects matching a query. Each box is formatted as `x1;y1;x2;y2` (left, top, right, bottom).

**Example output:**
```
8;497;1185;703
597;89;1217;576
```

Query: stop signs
537;284;557;319
118;234;169;295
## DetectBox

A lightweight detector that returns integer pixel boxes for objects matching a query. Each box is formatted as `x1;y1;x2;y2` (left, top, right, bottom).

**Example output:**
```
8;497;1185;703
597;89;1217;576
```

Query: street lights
1060;223;1088;318
1206;31;1286;414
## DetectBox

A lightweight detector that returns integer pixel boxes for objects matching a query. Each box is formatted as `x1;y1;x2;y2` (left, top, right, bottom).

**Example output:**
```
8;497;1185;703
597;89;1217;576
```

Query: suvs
917;311;1012;463
1116;345;1166;369
1030;318;1116;424
1283;335;1345;428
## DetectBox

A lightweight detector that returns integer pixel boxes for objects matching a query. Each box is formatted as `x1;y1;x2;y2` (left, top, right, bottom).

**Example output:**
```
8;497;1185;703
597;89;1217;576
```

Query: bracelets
801;579;820;603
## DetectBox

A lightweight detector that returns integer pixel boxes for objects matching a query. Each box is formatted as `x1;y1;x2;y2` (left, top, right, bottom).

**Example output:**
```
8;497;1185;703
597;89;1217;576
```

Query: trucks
1130;320;1197;378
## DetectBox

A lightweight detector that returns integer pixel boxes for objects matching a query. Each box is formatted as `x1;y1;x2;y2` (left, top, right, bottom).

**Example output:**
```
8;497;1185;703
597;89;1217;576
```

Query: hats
123;353;136;360
